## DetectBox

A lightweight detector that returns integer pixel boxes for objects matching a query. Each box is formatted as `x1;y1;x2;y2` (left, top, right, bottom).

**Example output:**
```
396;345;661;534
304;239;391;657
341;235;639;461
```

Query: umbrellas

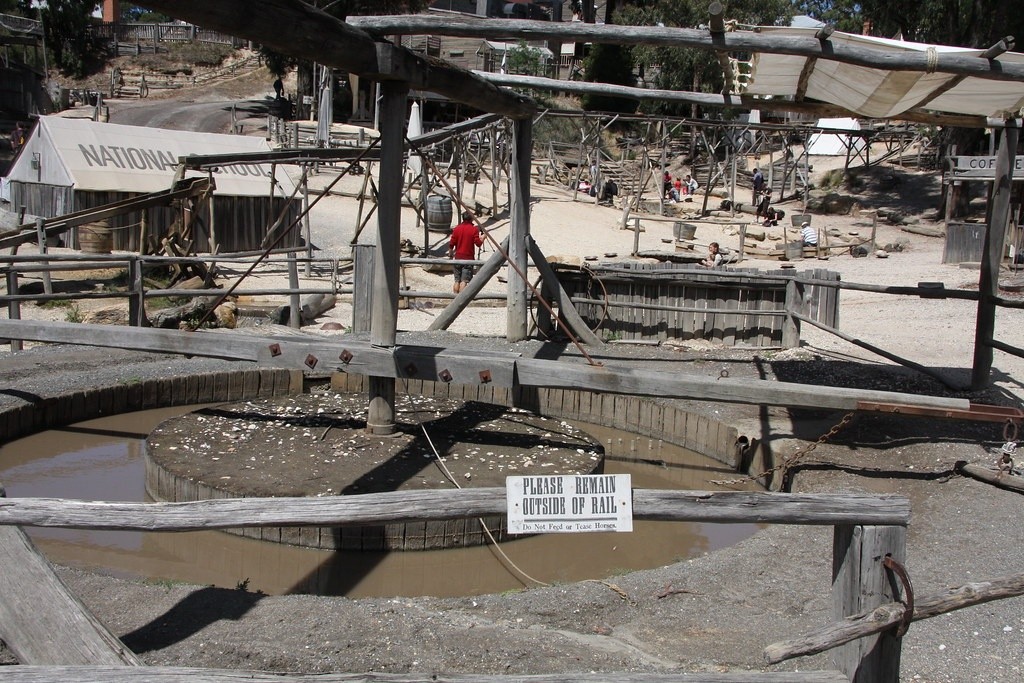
316;87;331;149
406;101;421;139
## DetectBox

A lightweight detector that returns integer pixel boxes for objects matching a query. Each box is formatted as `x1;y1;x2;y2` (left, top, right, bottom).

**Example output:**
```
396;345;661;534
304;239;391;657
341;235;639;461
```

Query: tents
803;117;867;156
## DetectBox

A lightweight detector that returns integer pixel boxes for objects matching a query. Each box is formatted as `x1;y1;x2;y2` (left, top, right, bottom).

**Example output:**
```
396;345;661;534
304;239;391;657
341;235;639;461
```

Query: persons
763;207;778;227
448;212;488;294
273;78;284;102
664;170;699;204
591;160;600;178
701;242;723;268
751;167;764;206
600;179;618;201
423;100;449;132
11;122;29;151
800;222;818;247
756;182;772;223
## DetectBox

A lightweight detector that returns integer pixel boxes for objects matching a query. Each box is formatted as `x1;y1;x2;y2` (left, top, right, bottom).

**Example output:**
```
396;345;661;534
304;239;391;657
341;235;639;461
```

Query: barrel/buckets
78;216;114;253
673;222;697;239
0;138;11;157
427;194;453;233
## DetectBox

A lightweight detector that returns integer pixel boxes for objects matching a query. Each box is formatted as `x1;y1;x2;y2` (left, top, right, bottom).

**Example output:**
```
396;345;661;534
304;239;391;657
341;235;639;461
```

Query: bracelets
449;255;452;257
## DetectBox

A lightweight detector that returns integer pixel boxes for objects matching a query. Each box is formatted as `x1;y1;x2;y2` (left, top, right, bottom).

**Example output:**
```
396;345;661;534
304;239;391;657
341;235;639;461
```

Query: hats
801;222;808;227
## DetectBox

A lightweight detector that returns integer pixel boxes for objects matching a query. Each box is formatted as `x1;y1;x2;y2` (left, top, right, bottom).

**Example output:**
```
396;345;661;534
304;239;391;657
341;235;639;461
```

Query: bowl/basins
964;219;978;223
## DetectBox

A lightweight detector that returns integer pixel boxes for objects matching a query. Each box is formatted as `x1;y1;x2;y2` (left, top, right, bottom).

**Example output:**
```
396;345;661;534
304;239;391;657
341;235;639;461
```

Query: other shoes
752;221;758;224
670;200;677;203
459;285;465;293
454;283;459;293
759;222;764;225
